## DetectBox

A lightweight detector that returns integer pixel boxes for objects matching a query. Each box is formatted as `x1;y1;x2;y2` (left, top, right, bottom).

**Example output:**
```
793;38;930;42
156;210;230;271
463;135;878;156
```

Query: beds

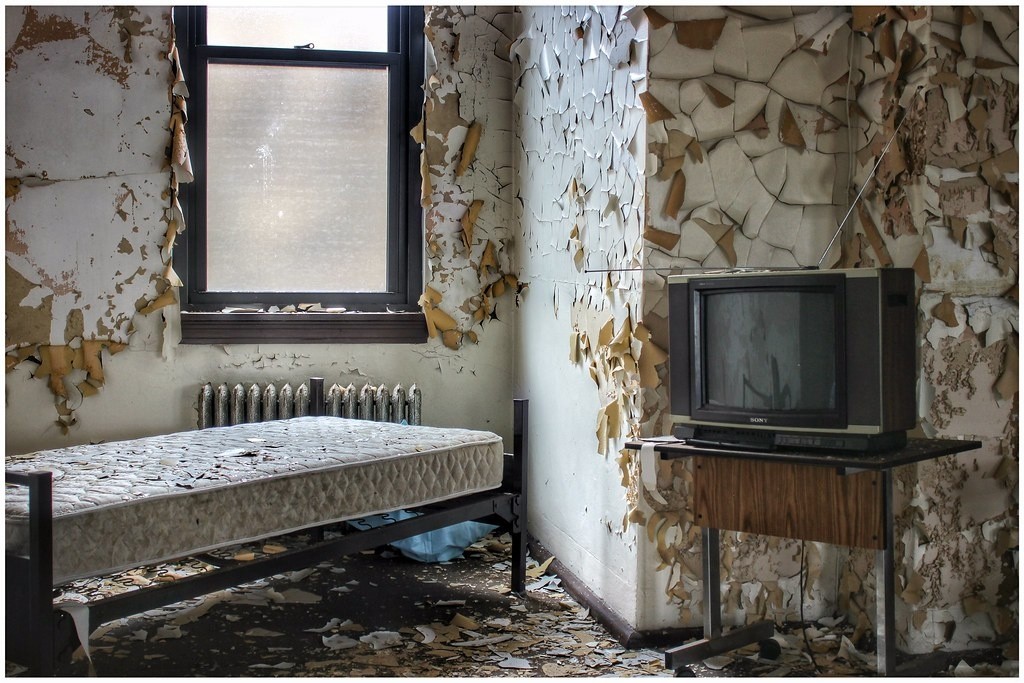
5;377;530;678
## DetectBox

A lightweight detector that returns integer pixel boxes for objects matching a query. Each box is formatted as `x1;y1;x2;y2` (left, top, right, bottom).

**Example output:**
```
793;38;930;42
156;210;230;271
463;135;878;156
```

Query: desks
624;437;1004;677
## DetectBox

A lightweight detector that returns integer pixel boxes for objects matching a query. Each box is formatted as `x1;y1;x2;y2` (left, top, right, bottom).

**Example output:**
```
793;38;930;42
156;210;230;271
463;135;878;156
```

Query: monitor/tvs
664;269;919;454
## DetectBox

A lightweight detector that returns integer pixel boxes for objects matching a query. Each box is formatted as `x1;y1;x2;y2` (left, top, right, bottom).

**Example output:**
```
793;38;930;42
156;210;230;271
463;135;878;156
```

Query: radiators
197;382;422;426
